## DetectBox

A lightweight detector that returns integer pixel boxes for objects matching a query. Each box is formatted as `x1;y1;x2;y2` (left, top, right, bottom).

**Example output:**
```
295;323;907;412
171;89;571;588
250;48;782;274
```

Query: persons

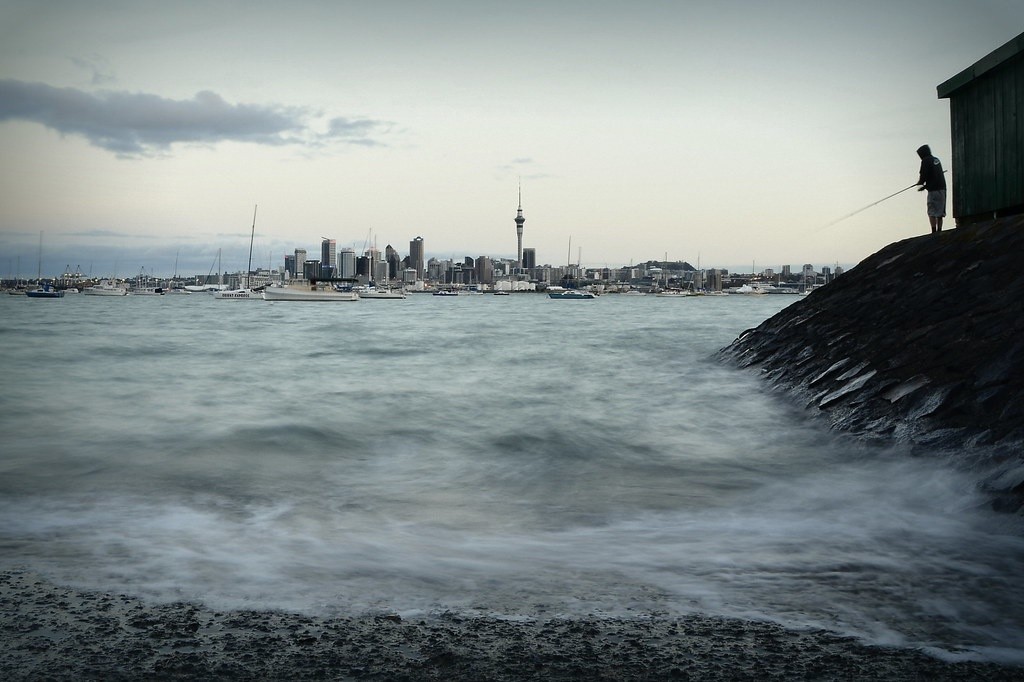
917;145;946;232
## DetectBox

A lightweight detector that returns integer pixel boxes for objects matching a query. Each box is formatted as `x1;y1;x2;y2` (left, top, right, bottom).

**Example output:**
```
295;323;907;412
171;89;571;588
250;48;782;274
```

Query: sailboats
208;205;265;300
8;232;191;298
184;247;228;292
350;227;406;298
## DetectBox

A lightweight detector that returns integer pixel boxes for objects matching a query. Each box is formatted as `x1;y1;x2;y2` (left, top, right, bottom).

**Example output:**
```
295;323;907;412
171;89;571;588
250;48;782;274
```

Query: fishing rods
828;169;949;223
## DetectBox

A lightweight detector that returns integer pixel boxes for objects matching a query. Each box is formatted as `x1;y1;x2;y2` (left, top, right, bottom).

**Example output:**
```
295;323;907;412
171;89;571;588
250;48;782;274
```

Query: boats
655;290;704;298
549;291;595;300
432;290;460;296
494;291;509;296
265;279;361;302
619;291;648;296
